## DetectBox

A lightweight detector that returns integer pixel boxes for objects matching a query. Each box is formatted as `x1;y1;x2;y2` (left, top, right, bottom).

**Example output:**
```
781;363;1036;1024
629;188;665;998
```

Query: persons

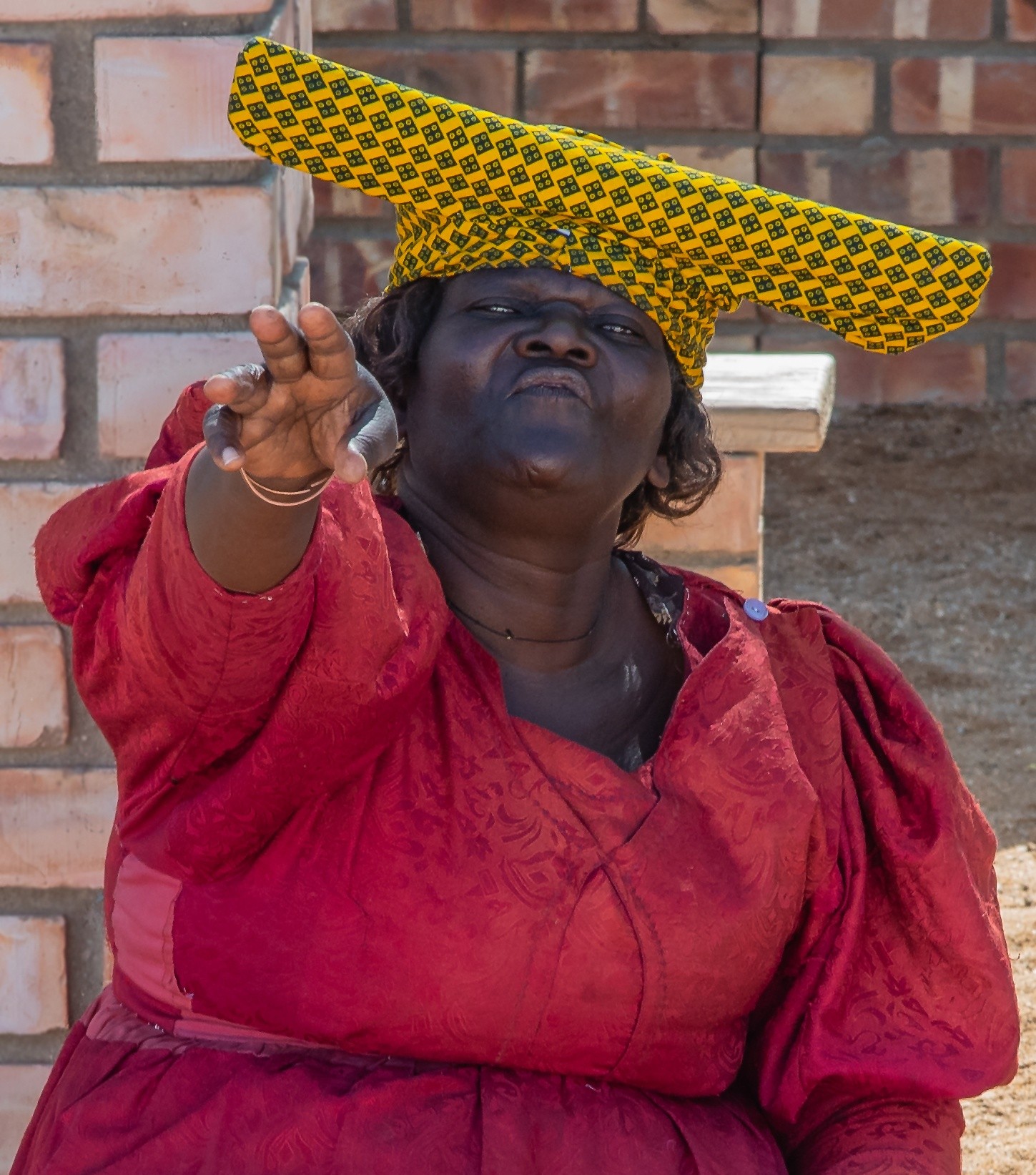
11;39;1020;1175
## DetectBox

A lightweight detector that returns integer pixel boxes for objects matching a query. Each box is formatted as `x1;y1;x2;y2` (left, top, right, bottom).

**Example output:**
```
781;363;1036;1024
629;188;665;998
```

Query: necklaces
448;597;598;643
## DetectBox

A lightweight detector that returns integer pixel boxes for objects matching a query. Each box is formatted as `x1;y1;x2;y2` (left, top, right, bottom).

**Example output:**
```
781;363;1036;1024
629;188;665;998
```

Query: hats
225;38;995;401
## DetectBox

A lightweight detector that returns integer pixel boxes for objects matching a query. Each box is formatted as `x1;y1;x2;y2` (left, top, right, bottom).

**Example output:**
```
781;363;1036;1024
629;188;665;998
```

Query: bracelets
240;466;335;506
